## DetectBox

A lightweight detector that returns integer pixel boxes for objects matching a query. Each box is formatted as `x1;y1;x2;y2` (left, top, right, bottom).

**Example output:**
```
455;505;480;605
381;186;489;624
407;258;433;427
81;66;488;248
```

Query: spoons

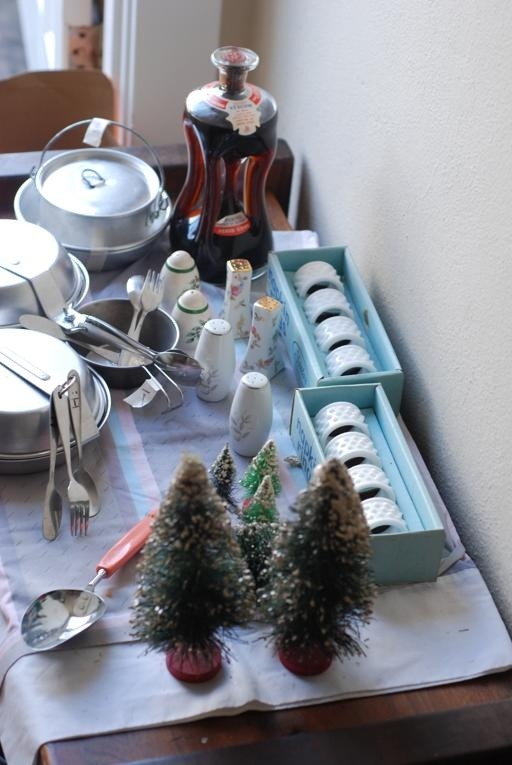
127;274;144;338
67;369;100;518
19;313;120;363
85;315;201;385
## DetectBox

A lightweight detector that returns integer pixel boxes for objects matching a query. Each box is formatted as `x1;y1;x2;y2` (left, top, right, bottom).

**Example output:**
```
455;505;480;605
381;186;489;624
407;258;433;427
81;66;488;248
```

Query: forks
133;268;165;341
53;384;90;537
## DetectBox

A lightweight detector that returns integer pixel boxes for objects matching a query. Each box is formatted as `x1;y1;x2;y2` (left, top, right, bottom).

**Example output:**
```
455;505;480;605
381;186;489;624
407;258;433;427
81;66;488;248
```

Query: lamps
1;69;119;153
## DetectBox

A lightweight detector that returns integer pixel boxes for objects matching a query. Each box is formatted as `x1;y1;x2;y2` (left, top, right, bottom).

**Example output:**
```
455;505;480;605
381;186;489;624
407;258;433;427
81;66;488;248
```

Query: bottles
238;298;283;382
219;259;253;341
194;319;236;401
230;371;272;456
169;47;275;285
159;250;200;325
171;291;210;356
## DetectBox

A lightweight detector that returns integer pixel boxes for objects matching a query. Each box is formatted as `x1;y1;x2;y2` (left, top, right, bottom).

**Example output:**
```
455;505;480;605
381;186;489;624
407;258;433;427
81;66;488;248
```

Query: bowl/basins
14;177;172;273
68;300;180;389
1;329;112;476
0;219;90;330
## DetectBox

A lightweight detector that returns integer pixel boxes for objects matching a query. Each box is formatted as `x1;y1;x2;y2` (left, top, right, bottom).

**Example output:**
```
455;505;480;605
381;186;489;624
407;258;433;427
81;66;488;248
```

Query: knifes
41;392;63;540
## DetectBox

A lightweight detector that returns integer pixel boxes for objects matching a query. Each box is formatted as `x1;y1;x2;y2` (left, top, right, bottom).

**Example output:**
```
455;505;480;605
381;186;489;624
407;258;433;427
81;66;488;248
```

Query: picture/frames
0;137;511;764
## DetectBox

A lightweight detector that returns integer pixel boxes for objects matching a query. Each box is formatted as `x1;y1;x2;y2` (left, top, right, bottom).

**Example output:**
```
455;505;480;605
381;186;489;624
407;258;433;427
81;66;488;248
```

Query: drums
41;392;63;540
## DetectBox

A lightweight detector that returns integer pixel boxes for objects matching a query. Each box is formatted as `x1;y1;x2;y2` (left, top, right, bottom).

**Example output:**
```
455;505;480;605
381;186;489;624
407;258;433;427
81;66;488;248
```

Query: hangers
19;313;120;363
127;274;144;338
85;315;201;385
67;369;100;518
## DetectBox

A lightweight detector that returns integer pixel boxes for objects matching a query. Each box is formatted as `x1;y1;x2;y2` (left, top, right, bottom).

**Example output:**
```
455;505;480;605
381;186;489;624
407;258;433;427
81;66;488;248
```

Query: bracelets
68;300;180;389
0;219;90;330
1;329;112;476
14;177;172;273
